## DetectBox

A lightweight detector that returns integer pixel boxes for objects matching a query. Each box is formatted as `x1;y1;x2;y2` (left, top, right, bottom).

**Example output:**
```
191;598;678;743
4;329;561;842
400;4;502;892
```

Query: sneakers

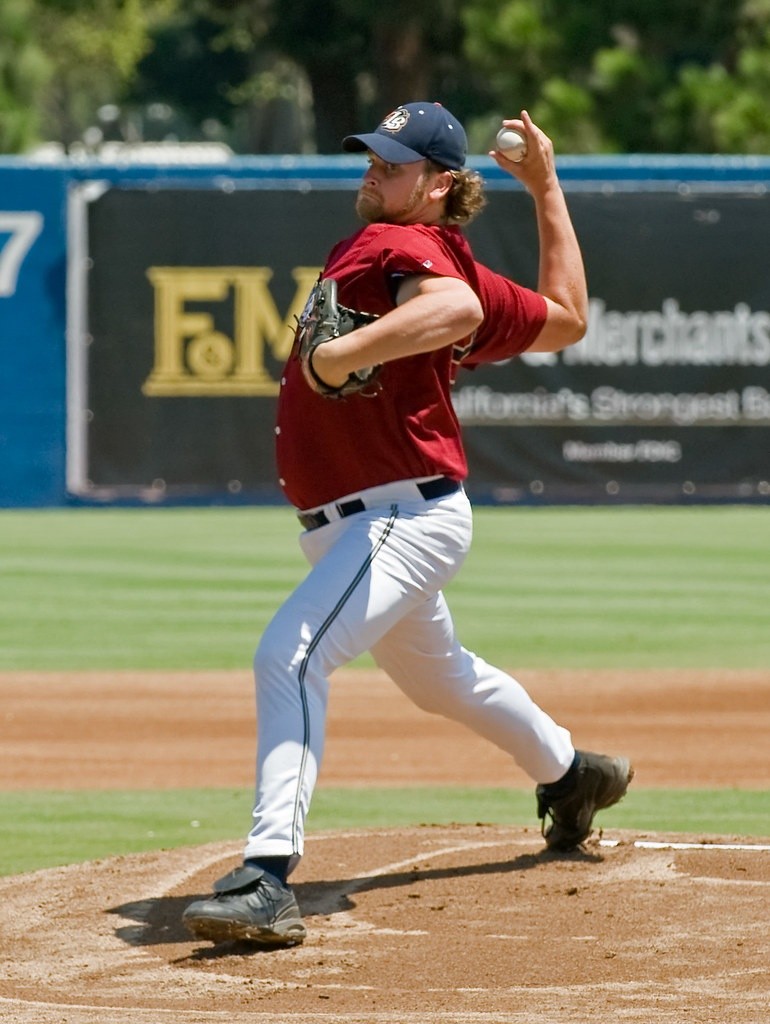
183;867;305;946
536;750;635;853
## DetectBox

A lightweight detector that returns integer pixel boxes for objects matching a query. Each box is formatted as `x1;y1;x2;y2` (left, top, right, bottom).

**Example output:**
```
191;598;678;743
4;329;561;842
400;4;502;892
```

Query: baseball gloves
300;278;374;397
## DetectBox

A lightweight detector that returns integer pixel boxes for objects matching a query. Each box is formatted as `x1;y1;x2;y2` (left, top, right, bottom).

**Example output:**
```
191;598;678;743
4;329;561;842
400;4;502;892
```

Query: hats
342;101;467;172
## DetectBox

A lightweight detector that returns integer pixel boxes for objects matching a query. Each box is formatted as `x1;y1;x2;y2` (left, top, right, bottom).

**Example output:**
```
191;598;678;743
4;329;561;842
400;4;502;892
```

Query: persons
181;102;635;946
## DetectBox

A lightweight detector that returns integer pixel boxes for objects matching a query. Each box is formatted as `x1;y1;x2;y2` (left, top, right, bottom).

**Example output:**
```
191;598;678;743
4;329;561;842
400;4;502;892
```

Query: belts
298;476;462;532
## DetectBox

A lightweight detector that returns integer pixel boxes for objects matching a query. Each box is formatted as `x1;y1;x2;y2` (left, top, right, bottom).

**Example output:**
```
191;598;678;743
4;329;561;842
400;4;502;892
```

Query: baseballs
496;127;527;163
